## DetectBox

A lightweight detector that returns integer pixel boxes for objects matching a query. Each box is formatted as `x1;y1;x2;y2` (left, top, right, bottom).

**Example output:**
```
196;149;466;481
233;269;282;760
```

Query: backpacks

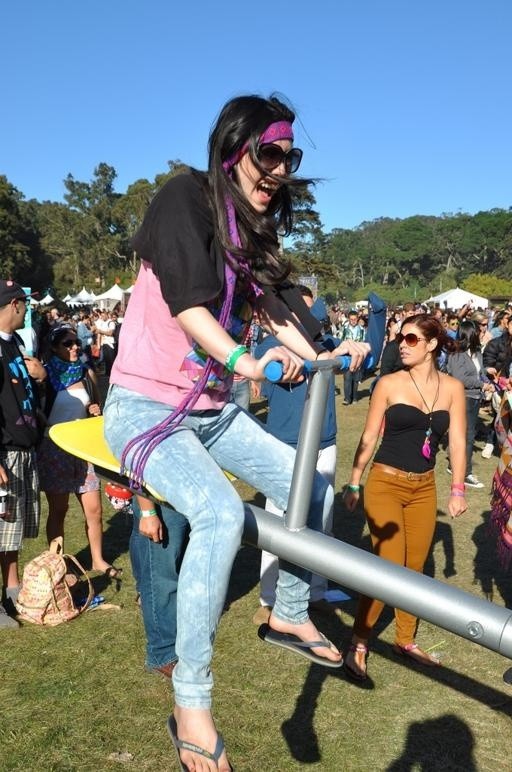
15;535;97;626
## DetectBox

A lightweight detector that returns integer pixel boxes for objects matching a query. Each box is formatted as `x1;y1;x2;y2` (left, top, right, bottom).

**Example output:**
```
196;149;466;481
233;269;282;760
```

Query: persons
232;283;512;677
100;94;373;771
1;282;192;676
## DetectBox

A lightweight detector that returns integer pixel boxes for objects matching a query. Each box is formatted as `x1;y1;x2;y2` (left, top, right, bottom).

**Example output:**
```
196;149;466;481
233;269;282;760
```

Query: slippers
166;710;233;772
258;622;344;669
90;561;124;579
344;640;368;680
392;640;442;668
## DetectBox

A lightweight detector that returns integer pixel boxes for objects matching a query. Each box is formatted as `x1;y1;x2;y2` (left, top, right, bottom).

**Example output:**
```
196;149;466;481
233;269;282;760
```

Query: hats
0;280;39;307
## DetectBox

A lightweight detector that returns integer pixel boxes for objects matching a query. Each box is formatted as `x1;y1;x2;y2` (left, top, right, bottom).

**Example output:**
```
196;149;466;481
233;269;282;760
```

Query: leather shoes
143;657;177;679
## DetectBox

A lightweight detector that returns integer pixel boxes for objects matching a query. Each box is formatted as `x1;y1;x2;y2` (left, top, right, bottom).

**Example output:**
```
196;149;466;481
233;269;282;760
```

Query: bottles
81;595;105;609
0;484;7;518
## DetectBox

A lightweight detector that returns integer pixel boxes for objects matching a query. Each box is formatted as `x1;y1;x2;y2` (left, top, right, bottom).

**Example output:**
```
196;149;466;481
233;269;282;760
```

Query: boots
1;583;24;614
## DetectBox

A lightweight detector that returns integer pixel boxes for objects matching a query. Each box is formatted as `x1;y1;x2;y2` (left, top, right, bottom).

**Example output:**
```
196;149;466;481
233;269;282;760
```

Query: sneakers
1;604;21;629
446;457;485;489
481;443;494;458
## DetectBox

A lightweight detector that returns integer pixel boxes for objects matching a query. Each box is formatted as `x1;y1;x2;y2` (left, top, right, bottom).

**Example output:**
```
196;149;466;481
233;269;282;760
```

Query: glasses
62;339;83;348
395;333;432;346
17;299;31;306
256;142;305;173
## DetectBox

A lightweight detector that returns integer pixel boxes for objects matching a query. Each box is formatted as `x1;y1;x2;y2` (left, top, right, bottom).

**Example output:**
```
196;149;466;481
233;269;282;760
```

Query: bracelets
224;344;247;373
